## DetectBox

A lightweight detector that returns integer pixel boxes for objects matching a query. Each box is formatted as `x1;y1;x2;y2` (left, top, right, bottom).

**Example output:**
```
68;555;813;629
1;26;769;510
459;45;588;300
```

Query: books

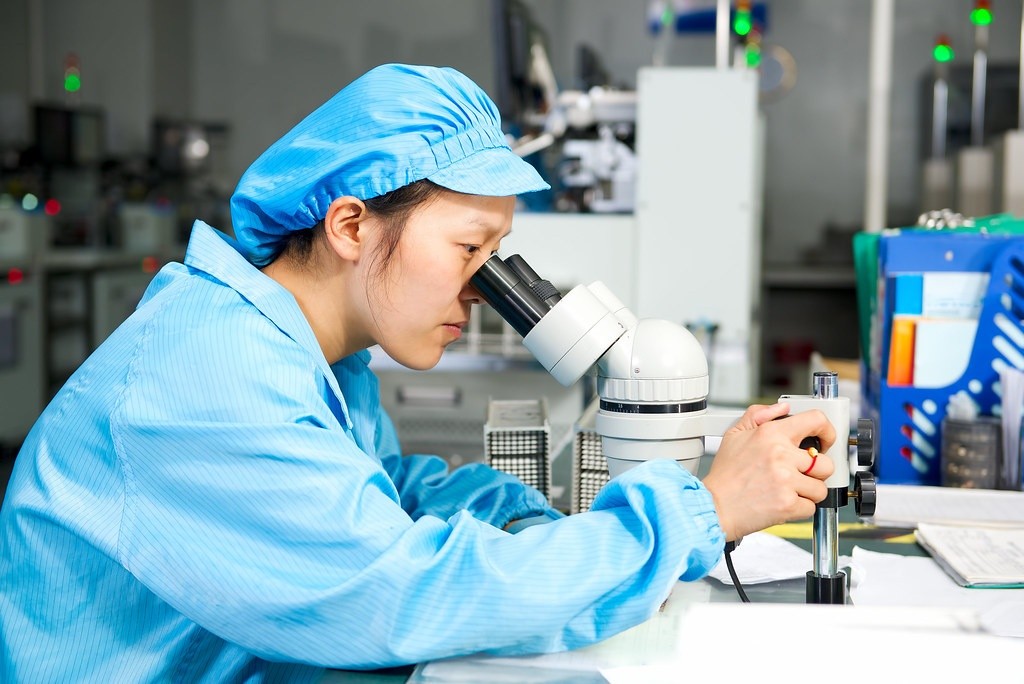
879;267;1024;474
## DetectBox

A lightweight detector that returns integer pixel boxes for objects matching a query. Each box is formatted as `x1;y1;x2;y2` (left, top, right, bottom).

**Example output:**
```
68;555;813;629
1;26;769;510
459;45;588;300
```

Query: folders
851;212;1024;379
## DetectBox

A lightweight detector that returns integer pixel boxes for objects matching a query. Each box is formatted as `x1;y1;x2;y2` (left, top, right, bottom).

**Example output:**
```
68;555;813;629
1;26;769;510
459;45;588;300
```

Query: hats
229;62;552;266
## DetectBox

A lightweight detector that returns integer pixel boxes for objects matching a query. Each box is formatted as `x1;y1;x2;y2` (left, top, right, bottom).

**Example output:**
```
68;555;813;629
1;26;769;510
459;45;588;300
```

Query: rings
801;448;818;474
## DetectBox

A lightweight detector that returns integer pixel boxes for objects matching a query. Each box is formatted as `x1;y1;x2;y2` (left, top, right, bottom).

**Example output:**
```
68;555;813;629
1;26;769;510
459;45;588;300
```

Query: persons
0;62;836;683
